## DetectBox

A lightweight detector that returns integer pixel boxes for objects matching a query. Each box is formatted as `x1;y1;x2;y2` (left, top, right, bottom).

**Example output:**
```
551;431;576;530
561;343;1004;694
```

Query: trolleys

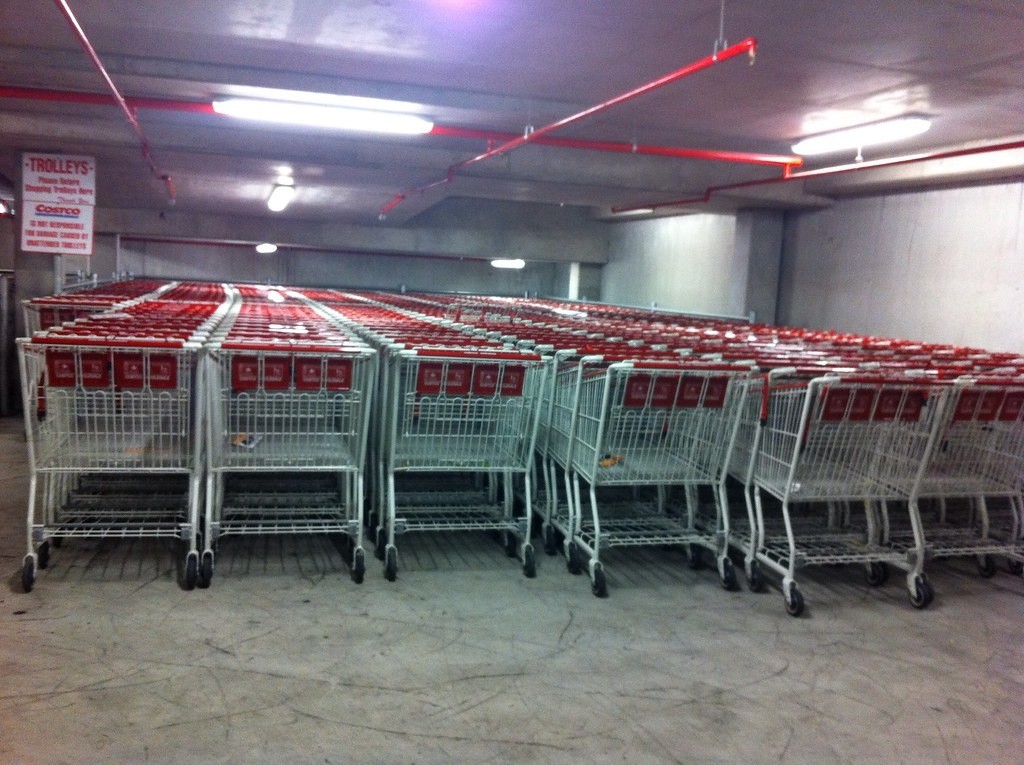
17;277;1023;626
